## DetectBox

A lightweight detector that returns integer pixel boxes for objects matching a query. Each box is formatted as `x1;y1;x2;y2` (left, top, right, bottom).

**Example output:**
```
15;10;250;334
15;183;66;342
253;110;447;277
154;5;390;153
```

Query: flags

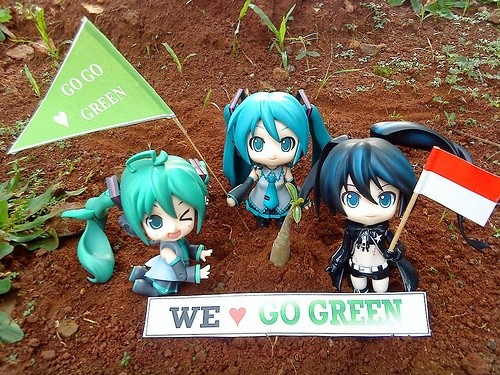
414;146;499;226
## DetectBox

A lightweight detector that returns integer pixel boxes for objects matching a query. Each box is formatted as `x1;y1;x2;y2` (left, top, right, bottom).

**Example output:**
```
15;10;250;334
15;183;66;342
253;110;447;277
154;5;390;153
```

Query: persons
222;86;334;230
59;149;213;298
270;120;492;294
